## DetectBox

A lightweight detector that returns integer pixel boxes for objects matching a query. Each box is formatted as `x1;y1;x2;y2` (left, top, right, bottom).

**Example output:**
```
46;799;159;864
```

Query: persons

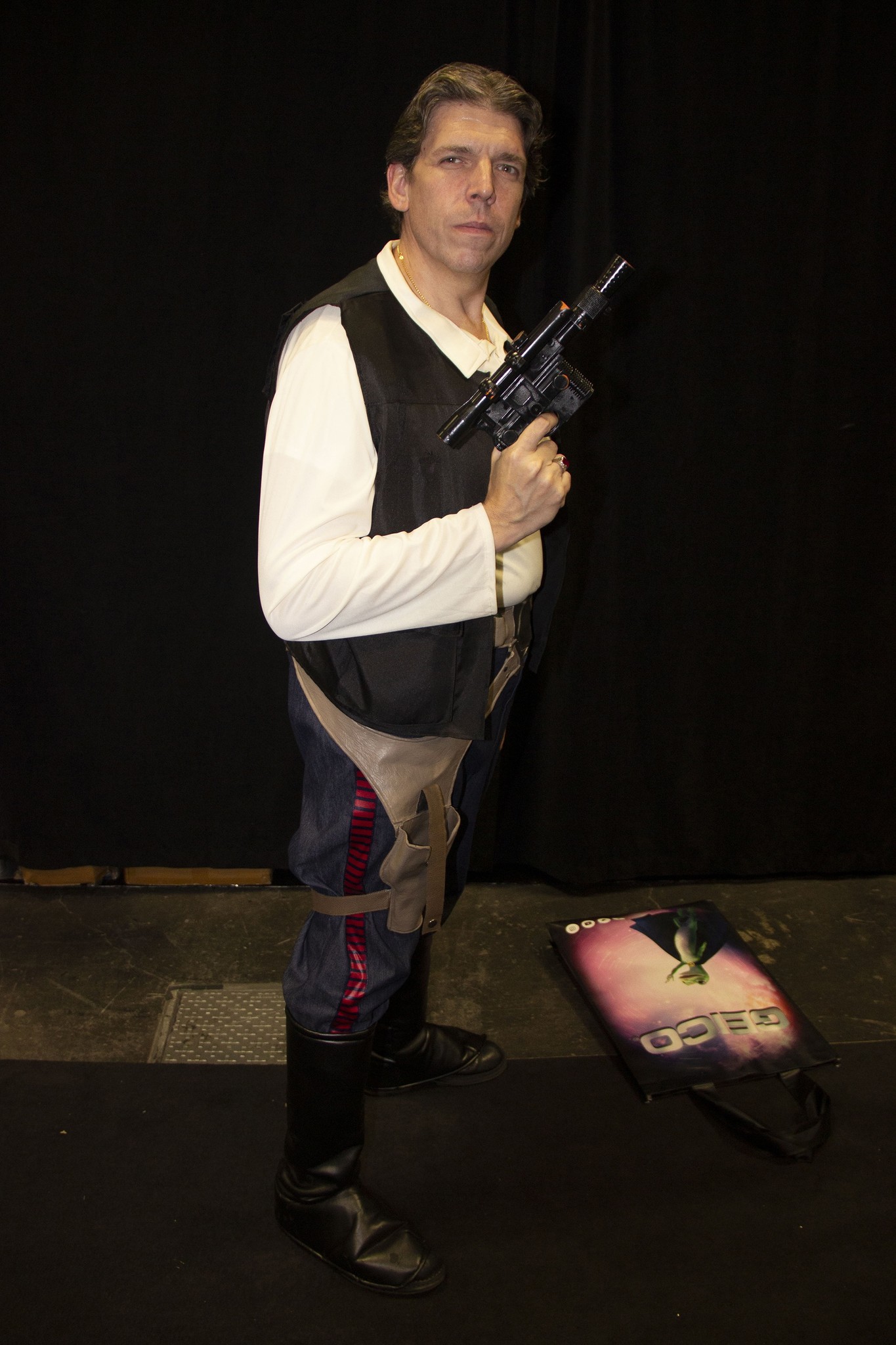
258;58;575;1299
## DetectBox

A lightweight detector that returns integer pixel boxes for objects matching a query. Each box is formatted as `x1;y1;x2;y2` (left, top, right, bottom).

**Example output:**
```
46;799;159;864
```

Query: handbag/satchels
549;898;843;1158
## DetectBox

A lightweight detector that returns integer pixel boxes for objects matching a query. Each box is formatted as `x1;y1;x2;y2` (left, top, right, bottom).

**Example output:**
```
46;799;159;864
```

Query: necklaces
396;240;490;343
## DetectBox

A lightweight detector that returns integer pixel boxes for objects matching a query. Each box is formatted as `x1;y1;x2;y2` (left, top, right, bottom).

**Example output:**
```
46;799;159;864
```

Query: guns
435;253;635;459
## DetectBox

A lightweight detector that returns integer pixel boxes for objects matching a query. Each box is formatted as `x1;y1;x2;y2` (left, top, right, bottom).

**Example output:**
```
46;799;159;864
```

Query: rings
553;456;569;473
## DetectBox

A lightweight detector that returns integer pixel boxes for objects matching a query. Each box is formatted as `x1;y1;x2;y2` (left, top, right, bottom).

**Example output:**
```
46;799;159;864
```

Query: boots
363;934;505;1099
278;1004;443;1295
